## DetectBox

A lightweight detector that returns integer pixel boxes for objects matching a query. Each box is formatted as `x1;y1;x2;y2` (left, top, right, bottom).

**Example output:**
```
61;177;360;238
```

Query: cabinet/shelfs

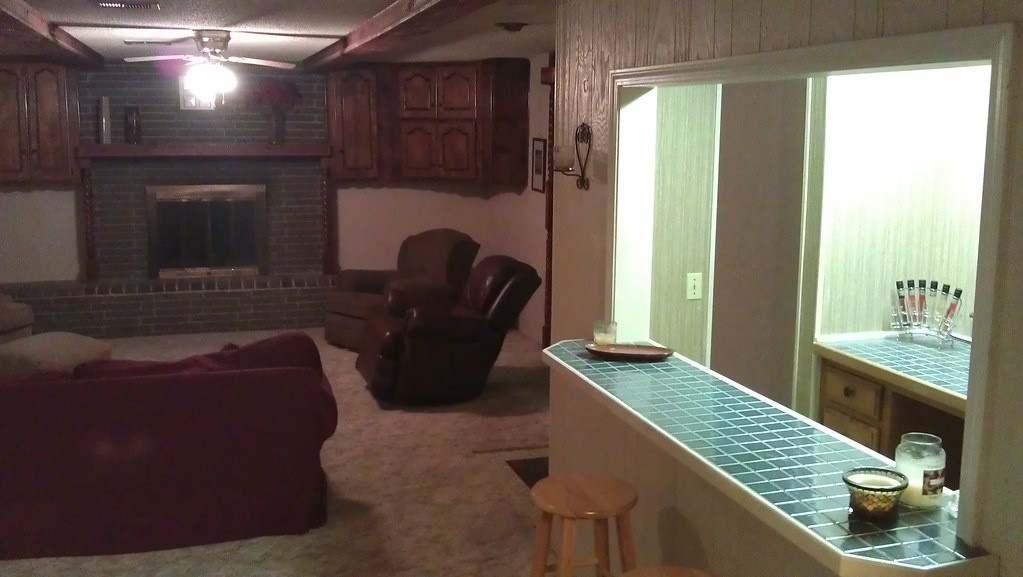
325;57;529;186
820;357;956;460
0;52;86;183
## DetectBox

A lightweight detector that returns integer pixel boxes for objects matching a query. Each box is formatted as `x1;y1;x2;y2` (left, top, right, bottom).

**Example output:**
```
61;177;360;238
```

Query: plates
585;344;674;360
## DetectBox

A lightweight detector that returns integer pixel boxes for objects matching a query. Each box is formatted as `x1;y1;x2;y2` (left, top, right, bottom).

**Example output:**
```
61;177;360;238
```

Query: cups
592;319;617;345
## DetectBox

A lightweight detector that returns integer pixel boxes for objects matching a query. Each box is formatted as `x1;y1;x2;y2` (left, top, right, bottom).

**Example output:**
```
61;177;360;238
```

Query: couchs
323;229;482;351
355;254;542;406
0;293;338;559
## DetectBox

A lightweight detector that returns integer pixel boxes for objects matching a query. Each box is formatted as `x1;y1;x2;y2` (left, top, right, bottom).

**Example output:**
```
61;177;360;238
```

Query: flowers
242;77;302;113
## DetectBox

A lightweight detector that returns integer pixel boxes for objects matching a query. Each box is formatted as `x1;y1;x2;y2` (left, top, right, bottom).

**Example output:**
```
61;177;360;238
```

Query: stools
529;469;640;577
623;563;717;576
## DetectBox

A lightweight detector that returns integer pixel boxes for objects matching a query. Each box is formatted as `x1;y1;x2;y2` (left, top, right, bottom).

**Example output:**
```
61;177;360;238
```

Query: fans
123;30;296;74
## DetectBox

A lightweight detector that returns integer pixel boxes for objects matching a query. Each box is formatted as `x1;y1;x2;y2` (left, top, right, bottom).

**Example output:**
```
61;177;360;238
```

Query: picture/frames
531;137;548;193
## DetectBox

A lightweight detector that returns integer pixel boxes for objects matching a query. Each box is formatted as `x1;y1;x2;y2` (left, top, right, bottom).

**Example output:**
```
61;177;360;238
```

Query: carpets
506;457;549;488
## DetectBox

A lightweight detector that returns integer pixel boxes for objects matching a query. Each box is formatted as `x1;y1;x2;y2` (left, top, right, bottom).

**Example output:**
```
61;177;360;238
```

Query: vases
265;111;286;144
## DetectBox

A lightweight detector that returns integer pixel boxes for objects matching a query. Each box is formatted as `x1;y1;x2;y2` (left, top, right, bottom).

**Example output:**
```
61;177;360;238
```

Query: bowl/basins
842;467;908;515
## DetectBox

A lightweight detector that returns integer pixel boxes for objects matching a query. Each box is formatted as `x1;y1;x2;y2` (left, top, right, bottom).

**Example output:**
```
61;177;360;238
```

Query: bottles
893;433;946;509
896;280;962;330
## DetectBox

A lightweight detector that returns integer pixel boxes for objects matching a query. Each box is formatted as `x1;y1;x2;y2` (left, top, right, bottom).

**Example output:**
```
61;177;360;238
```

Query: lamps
549;121;592;191
181;60;239;90
